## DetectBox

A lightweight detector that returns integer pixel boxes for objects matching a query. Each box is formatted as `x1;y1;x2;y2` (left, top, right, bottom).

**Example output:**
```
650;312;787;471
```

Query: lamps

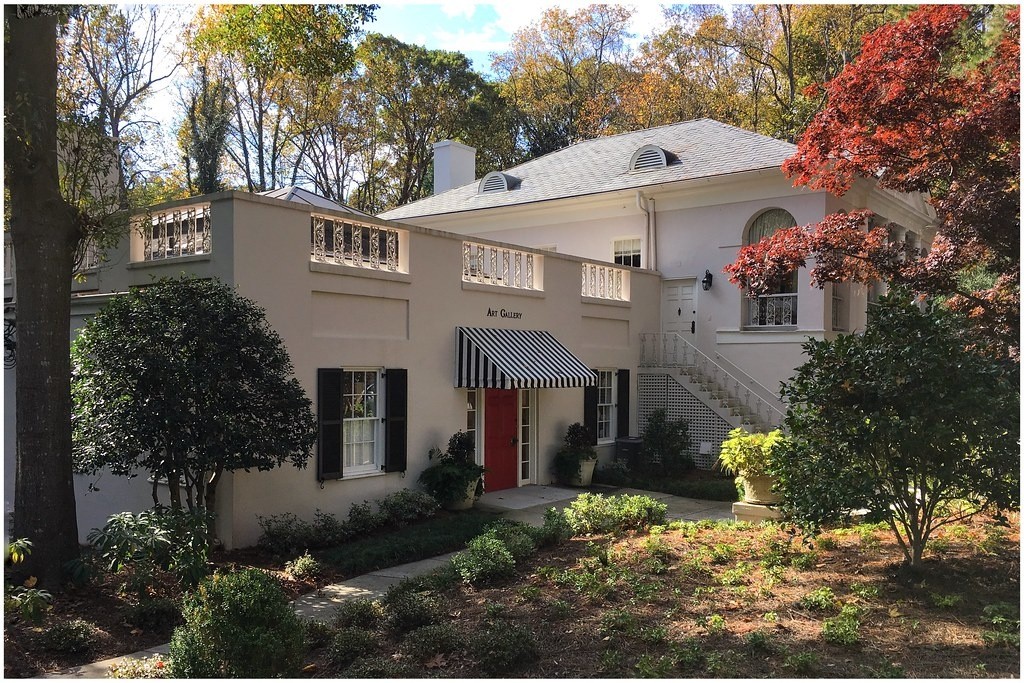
701;269;713;290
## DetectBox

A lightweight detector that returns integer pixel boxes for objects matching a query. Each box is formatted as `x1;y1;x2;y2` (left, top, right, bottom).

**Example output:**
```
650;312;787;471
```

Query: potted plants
717;426;783;506
550;421;597;485
420;428;484;511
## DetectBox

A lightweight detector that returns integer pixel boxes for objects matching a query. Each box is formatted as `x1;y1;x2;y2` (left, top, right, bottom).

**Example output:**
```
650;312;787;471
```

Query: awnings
453;326;599;390
